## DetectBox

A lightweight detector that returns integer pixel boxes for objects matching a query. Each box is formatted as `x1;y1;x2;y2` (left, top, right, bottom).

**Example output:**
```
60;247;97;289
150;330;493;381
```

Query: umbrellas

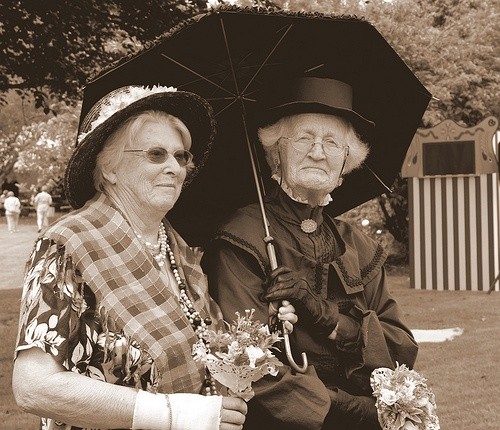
75;6;440;374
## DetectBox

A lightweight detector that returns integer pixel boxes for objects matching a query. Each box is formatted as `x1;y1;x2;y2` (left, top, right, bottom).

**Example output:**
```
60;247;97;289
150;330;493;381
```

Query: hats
62;83;217;209
263;74;378;137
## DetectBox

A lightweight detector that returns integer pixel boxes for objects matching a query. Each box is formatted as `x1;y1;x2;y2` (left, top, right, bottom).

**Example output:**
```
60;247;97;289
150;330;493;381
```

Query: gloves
259;266;339;336
326;386;382;430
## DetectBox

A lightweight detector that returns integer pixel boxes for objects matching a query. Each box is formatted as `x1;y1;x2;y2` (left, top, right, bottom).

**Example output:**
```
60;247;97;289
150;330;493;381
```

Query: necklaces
134;220;218;397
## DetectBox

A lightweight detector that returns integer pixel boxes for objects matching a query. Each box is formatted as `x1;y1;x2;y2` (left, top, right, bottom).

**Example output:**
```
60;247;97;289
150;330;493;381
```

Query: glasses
280;132;350;158
124;146;193;167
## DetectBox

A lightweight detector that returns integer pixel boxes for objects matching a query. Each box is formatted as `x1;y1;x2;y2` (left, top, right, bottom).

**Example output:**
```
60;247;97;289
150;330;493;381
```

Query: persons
12;85;248;430
0;190;21;233
207;78;421;430
33;185;53;233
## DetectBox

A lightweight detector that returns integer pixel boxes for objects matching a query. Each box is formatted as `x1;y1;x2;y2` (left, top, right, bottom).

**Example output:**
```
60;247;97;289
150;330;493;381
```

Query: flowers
192;308;284;392
371;361;440;430
